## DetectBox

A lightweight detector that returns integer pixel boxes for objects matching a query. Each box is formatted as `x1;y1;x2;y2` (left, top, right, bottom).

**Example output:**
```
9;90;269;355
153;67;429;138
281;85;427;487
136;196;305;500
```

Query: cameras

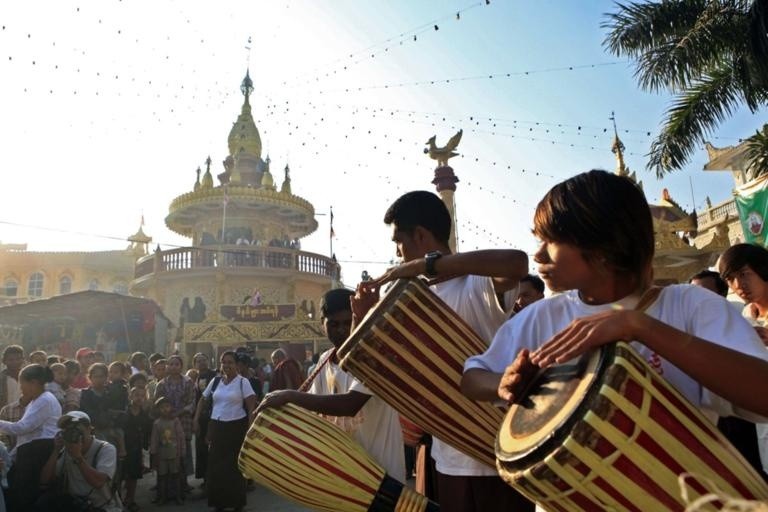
58;419;83;443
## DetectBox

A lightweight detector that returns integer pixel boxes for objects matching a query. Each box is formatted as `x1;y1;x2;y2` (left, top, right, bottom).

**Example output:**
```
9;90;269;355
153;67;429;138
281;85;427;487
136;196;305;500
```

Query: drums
237;395;444;512
493;337;764;511
337;277;512;472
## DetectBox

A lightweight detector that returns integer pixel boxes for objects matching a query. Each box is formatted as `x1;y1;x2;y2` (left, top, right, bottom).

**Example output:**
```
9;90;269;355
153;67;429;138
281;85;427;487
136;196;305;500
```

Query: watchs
424;249;442;276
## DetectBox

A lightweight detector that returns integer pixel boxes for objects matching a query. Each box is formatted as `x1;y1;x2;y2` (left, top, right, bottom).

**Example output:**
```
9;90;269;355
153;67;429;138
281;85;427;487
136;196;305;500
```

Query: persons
224;231;300;267
514;274;545;315
349;191;536;511
460;167;768;512
719;244;768;481
252;289;407;486
688;270;728;300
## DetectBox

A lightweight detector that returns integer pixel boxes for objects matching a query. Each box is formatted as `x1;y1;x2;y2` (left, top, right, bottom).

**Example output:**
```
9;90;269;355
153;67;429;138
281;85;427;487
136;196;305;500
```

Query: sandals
123;502;144;512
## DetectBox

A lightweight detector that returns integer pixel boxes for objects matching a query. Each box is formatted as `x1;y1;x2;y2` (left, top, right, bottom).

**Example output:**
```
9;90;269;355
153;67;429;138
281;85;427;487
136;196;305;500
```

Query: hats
75;347;95;360
57;410;91;429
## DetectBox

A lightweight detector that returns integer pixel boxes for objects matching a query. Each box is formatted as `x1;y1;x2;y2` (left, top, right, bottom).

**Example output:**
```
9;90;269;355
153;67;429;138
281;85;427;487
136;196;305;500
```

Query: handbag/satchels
199;393;214;438
